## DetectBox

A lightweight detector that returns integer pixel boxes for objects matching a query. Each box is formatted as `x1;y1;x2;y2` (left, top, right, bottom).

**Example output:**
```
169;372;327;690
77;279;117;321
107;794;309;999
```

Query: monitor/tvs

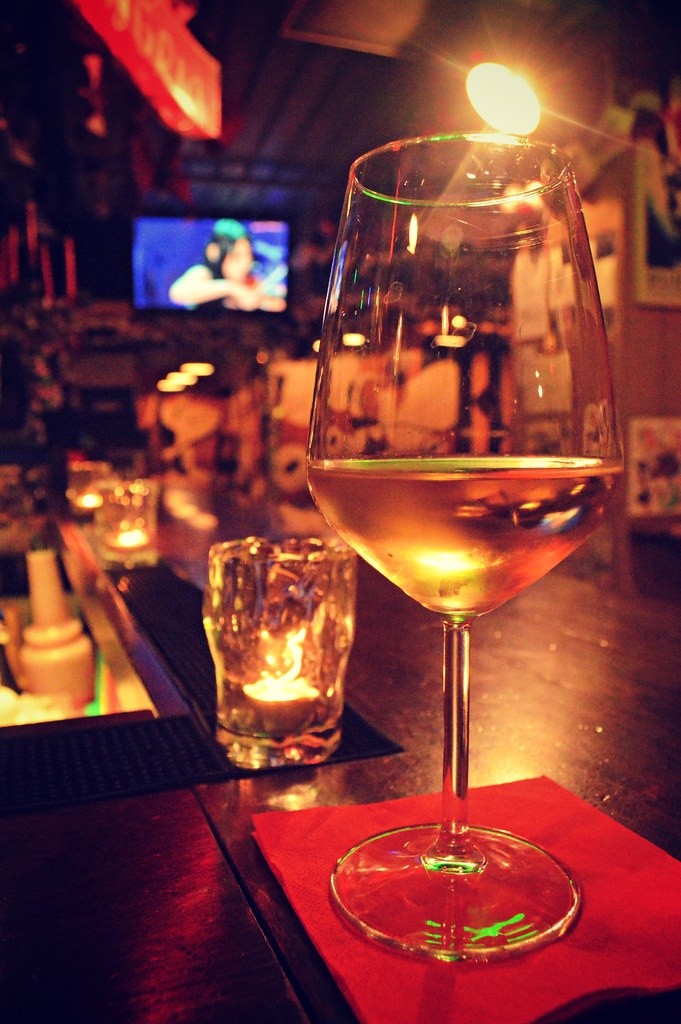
126;210;294;319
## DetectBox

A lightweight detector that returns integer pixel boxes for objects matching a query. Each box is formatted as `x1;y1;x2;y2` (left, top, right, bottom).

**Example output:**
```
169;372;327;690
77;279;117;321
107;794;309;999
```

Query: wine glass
304;132;626;962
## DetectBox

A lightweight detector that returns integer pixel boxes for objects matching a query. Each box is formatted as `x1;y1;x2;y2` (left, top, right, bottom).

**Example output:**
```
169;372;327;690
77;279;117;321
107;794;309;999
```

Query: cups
93;482;161;570
200;533;359;770
